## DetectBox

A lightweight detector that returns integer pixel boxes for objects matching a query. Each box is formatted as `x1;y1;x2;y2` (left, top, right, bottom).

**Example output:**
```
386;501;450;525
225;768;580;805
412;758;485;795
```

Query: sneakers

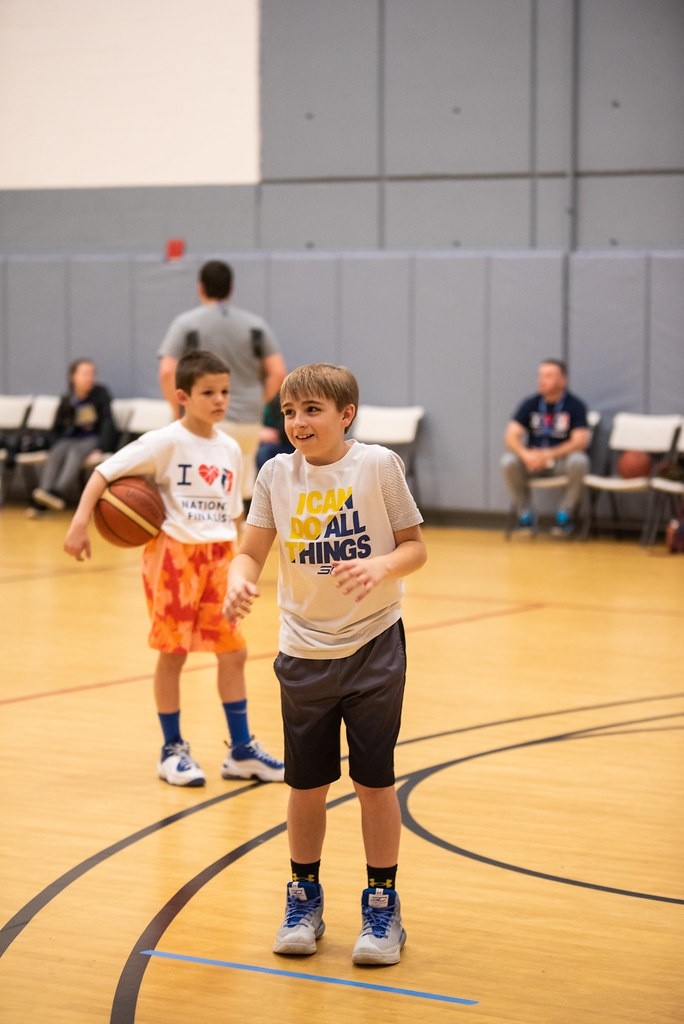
157;740;206;787
220;735;286;781
273;880;326;956
351;888;407;965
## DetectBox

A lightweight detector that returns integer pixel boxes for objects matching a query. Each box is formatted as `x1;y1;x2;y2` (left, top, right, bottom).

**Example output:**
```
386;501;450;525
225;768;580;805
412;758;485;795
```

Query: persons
224;361;427;968
157;260;294;532
501;358;592;535
27;356;120;521
64;350;328;788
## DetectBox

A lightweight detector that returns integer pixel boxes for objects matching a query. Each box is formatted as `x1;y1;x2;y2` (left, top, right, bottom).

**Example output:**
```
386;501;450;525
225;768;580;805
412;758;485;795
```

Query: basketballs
93;477;165;548
618;449;651;478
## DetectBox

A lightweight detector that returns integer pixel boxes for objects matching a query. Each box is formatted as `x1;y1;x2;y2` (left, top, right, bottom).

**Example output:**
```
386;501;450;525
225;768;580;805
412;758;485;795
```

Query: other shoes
552;523;571;537
517;511;536;539
33;489;66;510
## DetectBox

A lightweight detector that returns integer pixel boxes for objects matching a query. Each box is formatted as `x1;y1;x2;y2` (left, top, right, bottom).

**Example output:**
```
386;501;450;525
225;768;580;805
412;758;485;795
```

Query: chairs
0;393;425;516
502;410;684;547
